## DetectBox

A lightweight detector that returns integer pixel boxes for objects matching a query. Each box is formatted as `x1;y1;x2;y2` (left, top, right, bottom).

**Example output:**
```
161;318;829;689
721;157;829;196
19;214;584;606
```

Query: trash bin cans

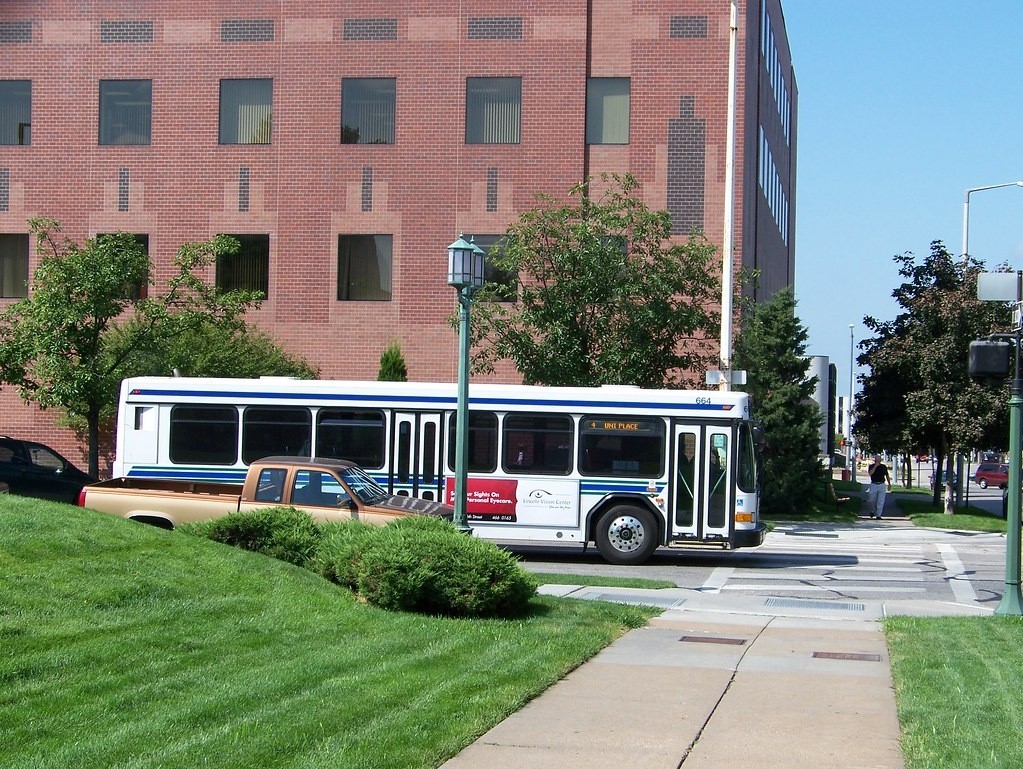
841;470;850;481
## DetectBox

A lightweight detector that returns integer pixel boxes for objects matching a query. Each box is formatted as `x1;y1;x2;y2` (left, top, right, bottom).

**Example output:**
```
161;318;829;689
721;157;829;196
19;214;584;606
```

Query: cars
915;453;928;464
929;470;959;491
0;435;103;509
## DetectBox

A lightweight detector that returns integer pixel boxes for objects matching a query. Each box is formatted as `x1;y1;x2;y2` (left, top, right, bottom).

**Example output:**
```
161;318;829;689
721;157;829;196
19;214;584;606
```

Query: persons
868;456;891;520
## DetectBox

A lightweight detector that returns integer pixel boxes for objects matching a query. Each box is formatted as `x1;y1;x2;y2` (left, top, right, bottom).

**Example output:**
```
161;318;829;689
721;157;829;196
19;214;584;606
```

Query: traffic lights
839;440;846;446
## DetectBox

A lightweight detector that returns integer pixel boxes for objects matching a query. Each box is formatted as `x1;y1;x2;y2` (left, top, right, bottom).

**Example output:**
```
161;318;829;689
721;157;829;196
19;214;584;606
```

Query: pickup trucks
79;455;458;540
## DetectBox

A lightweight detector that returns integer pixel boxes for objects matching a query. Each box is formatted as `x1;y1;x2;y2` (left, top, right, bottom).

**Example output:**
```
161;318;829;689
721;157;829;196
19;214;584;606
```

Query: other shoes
876;517;882;520
870;513;874;517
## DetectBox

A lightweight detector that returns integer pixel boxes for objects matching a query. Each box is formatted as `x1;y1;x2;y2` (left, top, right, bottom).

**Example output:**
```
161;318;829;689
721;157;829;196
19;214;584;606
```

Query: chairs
302;485;314;504
294;489;306;503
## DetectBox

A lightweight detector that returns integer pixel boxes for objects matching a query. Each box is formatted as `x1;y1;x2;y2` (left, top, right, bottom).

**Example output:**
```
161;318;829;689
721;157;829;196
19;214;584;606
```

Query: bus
112;372;766;567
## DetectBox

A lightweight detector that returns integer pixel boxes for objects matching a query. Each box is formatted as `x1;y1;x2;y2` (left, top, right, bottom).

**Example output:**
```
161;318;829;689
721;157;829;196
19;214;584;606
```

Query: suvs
975;462;1010;489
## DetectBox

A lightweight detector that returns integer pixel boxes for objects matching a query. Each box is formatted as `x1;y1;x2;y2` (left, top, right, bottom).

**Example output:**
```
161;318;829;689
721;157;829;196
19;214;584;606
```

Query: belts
872;482;883;485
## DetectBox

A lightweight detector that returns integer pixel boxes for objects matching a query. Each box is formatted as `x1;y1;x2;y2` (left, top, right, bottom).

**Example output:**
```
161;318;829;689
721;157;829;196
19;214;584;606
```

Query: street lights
958;182;1023;271
845;324;855;472
447;227;486;532
